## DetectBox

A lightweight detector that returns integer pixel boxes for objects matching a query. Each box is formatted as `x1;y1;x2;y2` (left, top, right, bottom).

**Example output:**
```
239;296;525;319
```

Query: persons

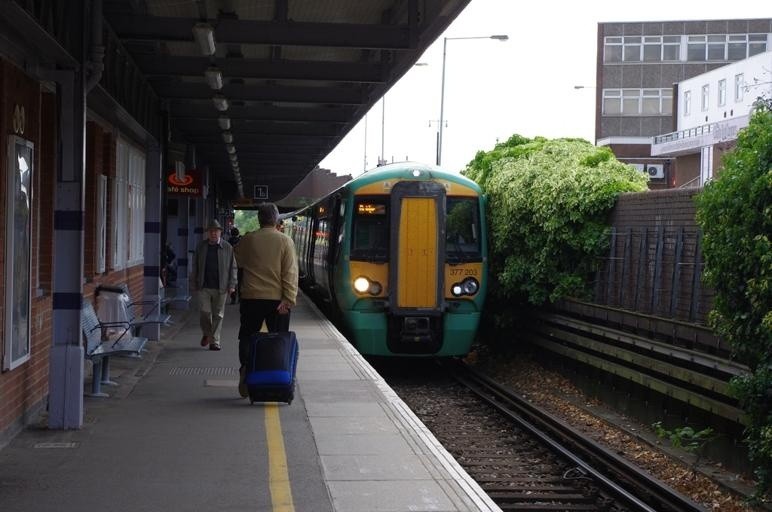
189;220;236;350
232;202;298;400
229;228;241;305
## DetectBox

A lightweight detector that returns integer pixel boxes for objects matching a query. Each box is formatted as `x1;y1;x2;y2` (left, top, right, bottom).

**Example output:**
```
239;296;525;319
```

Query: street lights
379;62;427;168
434;34;511;164
573;85;596;90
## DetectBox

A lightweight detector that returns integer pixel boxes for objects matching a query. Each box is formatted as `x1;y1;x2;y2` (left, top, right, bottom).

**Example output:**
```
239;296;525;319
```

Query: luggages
244;307;300;407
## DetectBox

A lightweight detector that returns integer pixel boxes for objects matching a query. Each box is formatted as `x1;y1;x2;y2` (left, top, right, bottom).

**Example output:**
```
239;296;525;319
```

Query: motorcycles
161;240;179;286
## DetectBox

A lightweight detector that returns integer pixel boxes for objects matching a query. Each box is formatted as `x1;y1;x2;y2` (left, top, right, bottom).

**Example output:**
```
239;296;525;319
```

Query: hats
230;227;239;237
205;219;224;232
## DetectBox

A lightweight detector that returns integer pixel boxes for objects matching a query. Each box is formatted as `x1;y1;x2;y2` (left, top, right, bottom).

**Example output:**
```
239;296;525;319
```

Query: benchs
114;283;167;344
158;278;194;327
81;300;148;400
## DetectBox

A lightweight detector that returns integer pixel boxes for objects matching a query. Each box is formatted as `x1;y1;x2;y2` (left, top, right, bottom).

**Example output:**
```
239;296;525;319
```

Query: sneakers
200;335;221;351
230;294;237;305
239;365;250;400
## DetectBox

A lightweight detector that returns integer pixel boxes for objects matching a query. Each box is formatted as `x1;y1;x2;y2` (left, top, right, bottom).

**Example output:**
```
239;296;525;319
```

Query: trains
275;161;487;364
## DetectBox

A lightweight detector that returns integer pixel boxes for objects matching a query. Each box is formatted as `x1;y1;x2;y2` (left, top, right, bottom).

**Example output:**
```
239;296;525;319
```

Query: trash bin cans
95;284;129;336
